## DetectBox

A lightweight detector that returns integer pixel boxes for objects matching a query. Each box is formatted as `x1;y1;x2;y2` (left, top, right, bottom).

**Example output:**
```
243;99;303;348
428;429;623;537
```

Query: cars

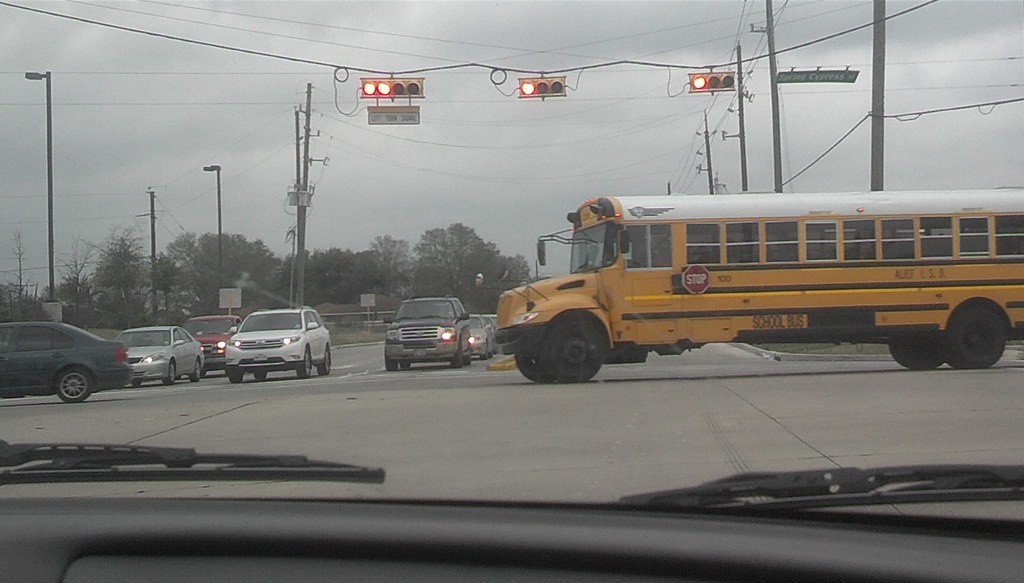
470;314;499;360
0;322;136;406
112;326;206;388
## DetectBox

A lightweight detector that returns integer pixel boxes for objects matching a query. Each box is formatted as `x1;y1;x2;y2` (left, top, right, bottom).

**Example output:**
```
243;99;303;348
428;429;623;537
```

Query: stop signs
682;263;712;295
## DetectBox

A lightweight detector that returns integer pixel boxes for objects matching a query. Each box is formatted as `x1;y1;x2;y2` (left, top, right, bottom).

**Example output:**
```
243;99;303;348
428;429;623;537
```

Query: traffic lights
519;76;569;98
358;77;426;99
688;71;737;93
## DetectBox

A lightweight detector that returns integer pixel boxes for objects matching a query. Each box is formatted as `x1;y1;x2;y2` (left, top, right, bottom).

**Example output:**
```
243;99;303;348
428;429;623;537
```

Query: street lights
25;70;61;302
203;164;224;305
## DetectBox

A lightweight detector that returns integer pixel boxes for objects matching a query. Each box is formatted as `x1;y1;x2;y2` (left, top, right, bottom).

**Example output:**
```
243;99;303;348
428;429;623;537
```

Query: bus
495;188;1023;379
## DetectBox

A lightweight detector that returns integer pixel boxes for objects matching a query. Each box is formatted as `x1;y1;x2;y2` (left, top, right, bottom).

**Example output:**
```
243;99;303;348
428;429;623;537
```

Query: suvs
224;305;332;384
382;293;471;368
180;314;243;377
